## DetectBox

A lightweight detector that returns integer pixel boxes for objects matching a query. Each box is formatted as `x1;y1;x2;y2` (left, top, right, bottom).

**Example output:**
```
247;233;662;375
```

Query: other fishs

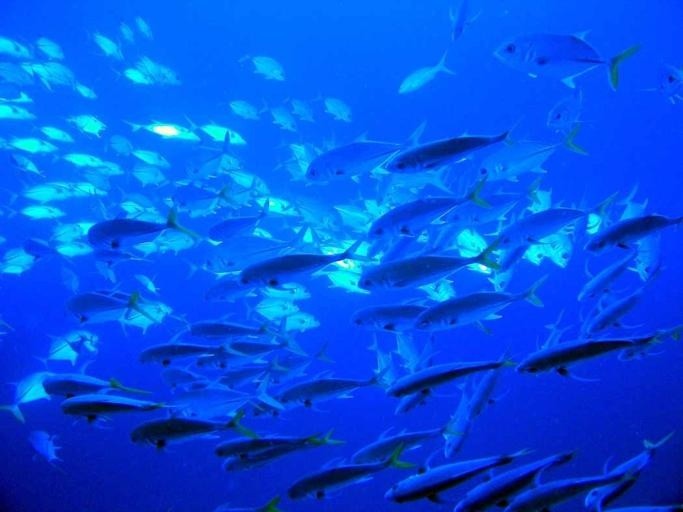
0;91;683;510
